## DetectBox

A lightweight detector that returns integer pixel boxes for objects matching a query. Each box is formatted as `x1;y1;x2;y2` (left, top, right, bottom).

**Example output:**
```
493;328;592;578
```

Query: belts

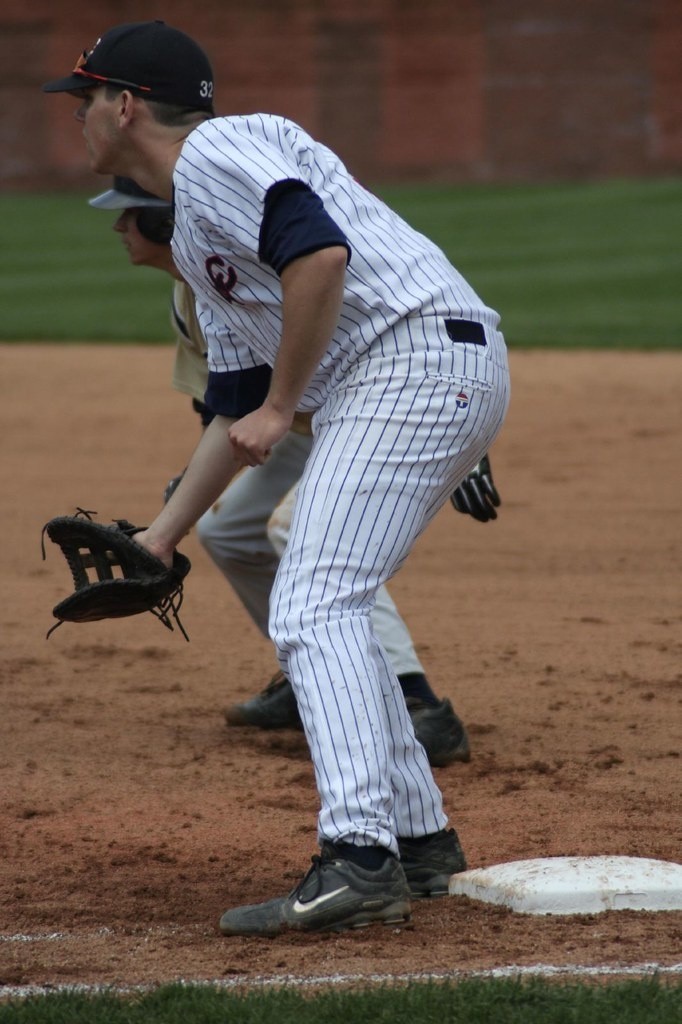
443;319;487;346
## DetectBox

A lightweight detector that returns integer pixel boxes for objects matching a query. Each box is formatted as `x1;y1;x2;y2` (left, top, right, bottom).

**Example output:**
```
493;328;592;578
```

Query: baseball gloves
39;515;195;624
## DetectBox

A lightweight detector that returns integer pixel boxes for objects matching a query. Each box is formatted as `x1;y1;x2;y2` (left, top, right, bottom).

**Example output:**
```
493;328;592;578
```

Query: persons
44;18;512;934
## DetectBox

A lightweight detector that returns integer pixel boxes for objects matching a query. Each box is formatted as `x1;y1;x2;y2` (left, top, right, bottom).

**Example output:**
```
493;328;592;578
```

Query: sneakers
225;669;298;727
402;694;471;767
397;826;468;898
219;841;411;935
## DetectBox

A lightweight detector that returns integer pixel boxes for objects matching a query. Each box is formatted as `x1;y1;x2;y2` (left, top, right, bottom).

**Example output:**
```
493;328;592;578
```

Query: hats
88;175;172;210
41;19;214;110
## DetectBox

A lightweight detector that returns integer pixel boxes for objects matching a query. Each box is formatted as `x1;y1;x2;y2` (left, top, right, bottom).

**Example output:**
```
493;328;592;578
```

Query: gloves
450;453;501;523
